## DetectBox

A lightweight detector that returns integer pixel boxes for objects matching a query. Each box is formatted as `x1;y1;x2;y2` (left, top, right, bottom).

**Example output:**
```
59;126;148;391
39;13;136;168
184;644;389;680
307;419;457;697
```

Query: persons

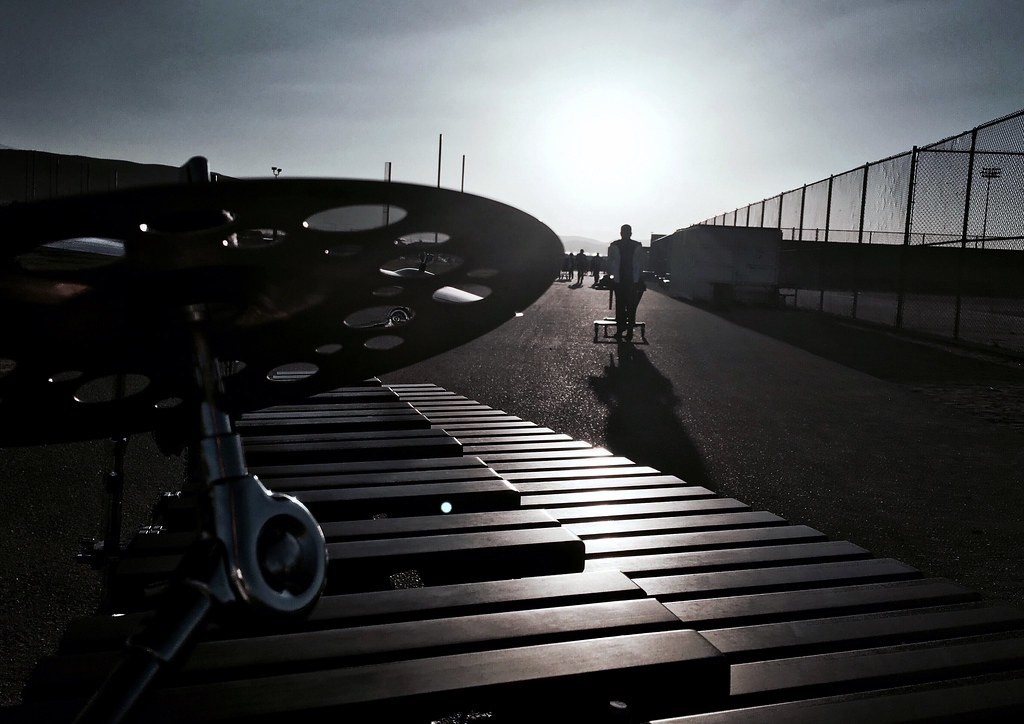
565;248;602;285
606;224;647;341
